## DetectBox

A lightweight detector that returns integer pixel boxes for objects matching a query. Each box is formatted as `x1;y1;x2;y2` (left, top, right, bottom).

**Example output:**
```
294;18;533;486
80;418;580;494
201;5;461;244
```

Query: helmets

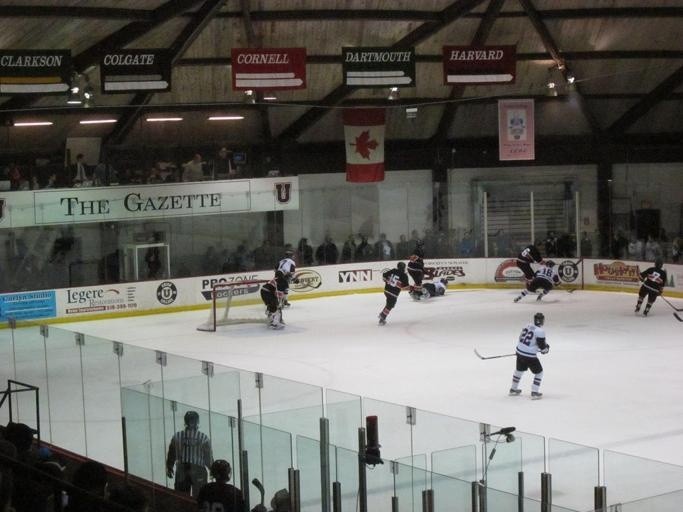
183;410;200;426
209;459;232;480
534;312;545;327
397;261;406;272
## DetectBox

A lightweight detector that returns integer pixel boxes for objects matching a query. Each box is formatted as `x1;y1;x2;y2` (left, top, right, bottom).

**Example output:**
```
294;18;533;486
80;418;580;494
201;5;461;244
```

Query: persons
514;261;560;302
4;230;30;262
164;410;215;496
635;256;667;316
411;282;445;301
611;224;683;264
1;146;232;191
199;235;277;278
287;226;480;263
406;240;426;284
198;456;242;512
379;262;408;326
1;422;151;511
543;229;592;258
277;251;296;304
516;240;545;287
261;270;289;327
510;312;549;397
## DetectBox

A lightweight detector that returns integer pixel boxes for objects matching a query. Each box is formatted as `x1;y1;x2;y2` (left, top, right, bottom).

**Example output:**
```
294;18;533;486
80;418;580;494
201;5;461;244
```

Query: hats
1;422;38;435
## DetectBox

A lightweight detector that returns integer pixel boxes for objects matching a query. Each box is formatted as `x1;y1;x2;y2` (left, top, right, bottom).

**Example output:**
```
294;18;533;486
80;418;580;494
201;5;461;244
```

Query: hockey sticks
559;284;576;293
661;295;683;311
299;280;322;288
673;313;683;322
474;348;517;359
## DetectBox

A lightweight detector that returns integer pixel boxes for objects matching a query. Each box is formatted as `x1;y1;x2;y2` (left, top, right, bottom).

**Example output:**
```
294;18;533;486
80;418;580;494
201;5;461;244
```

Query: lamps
66;72;93;105
262;90;277;100
546;71;575;97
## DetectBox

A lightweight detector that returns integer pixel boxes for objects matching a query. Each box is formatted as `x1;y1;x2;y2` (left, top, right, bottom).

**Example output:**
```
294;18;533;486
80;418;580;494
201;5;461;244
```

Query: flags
342;98;385;185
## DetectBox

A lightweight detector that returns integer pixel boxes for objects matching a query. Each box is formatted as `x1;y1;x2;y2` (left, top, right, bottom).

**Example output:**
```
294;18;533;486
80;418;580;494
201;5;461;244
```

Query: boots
509;388;522;393
633;306;648;317
530;391;543;397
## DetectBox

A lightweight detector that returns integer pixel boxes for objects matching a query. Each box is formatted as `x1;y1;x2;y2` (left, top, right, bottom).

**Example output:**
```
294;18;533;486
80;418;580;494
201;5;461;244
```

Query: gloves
166;464;175;479
